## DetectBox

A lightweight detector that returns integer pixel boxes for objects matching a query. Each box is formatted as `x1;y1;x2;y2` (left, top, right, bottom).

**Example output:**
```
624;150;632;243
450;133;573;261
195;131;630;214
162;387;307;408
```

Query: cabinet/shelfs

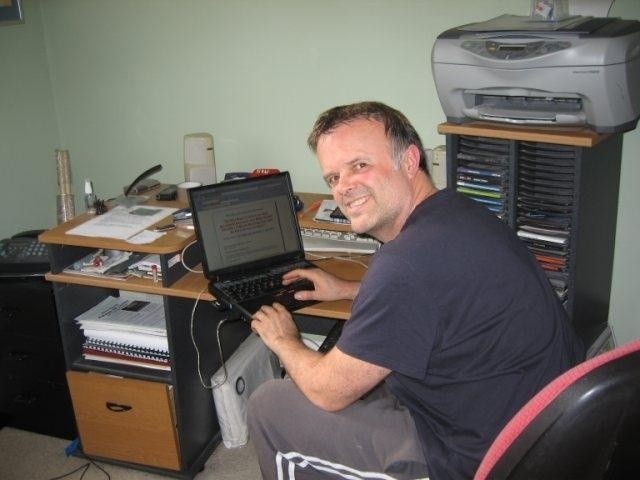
433;110;621;355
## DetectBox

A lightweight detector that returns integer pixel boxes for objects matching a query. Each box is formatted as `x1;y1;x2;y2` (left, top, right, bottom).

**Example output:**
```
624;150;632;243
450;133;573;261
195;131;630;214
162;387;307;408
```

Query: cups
177;181;202;207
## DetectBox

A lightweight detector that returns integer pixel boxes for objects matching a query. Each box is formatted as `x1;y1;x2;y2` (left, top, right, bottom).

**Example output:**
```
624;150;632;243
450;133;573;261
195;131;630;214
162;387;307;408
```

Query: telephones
0;230;51;272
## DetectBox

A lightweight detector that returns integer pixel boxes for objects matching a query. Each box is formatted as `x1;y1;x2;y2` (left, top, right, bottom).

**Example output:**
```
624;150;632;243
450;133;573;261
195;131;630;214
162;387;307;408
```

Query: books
75;290;171;374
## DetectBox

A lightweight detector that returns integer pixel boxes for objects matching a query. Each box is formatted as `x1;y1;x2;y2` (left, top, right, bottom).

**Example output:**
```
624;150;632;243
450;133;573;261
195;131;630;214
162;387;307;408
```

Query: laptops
185;170;336;328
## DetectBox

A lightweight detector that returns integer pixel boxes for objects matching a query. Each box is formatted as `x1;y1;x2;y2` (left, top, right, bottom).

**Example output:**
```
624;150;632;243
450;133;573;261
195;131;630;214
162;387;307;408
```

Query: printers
429;15;640;136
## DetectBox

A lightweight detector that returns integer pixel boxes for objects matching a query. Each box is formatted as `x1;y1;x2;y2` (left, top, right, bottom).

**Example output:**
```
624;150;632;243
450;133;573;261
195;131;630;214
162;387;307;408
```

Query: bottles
182;132;217;185
84;179;98;213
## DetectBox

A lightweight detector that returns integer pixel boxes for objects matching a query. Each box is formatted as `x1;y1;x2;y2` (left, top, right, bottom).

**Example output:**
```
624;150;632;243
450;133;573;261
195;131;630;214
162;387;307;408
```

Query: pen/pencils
299;200;321;218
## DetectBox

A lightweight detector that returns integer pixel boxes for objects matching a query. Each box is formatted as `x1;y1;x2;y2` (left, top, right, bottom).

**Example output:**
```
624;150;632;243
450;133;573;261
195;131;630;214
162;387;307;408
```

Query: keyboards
299;227;382;255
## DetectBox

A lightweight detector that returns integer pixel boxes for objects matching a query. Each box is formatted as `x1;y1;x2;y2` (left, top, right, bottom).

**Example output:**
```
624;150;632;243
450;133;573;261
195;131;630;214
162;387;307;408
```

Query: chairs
469;339;640;479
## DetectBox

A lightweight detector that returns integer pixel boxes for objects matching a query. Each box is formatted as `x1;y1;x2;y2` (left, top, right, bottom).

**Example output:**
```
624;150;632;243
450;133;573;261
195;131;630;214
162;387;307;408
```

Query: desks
33;182;384;478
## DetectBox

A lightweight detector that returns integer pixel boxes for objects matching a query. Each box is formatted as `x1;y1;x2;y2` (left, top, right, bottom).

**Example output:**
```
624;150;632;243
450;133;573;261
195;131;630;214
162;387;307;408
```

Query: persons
245;101;589;480
